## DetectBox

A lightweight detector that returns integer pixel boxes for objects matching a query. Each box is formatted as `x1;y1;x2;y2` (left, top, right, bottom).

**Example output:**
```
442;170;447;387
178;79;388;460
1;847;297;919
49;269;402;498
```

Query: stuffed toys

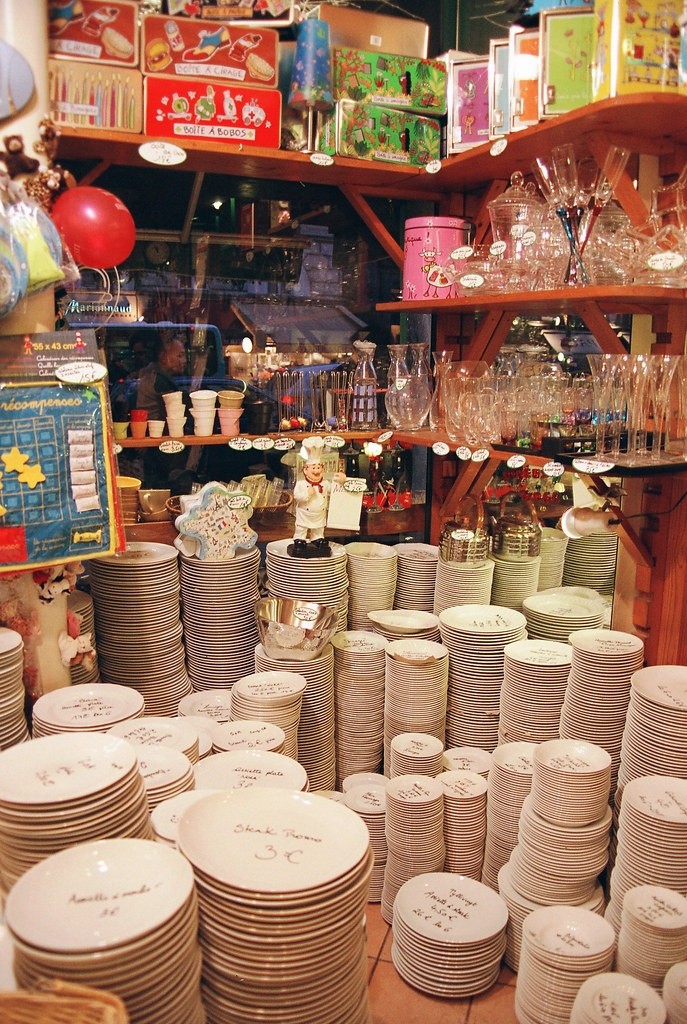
60;613;95;670
35;561;85;604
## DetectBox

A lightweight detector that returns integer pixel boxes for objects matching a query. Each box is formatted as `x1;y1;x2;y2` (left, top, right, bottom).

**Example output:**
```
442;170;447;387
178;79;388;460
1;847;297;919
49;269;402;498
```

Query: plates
0;538;687;1024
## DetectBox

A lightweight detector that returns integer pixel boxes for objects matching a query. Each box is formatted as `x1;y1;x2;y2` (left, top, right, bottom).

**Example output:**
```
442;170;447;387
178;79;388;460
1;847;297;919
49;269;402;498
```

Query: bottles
352;341;379;431
387;344;453;432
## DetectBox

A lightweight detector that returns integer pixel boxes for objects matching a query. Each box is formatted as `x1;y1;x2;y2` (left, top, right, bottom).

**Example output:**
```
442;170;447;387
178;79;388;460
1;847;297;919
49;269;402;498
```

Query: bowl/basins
116;477;141;525
253;597;340;661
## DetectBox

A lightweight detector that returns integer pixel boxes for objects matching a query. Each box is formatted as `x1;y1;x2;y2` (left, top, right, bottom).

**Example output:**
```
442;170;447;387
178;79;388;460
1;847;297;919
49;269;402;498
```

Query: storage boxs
47;0;687;166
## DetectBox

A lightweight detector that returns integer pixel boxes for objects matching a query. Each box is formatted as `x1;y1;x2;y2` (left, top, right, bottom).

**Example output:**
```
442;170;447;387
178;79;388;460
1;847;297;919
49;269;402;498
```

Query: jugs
437;493;490;569
489;491;543;563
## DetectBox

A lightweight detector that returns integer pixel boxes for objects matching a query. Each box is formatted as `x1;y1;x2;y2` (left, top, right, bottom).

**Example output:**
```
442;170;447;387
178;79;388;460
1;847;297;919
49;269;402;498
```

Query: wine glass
366;456;385;513
586;354;686;466
389;456;405;511
530;143;631;288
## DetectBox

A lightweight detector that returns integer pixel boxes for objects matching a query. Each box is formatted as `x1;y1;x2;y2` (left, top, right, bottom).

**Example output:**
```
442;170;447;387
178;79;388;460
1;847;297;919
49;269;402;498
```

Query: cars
265;362;348;421
109;376;317;497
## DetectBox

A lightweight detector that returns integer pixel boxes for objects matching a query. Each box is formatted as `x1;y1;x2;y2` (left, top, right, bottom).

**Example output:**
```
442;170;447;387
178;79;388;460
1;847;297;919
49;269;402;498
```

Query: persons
293;437;346;548
135;339;220;486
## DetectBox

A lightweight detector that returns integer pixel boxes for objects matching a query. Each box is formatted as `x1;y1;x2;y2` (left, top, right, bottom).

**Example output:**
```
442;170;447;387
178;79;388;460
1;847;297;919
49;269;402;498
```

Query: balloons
52;186;137;269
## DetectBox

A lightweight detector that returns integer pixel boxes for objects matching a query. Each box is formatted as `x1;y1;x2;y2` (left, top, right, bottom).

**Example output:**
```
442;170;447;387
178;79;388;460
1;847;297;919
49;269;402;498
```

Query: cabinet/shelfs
46;93;685;669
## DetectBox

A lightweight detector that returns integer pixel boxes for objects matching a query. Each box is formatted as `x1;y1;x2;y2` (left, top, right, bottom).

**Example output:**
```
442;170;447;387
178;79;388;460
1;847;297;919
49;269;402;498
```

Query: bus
64;319;228;387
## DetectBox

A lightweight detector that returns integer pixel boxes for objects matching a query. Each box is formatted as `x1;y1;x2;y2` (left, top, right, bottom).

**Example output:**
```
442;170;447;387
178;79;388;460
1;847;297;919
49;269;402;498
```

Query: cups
218;390;245;435
346;454;359;477
246;400;275;435
148;419;166;438
162;390;187;437
191;478;284;507
189;390;218;436
137;490;171;514
112;422;130;439
130;409;148;440
445;381;588;448
